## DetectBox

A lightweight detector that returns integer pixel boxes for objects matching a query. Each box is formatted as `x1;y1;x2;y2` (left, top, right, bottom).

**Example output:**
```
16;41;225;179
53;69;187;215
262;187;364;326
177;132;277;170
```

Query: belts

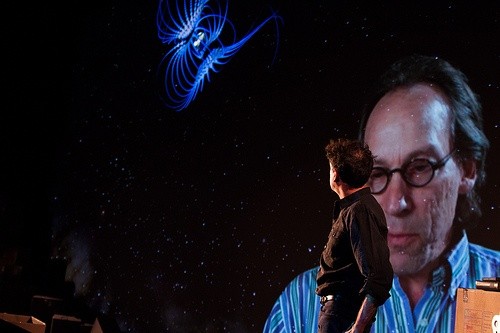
320;295;347;305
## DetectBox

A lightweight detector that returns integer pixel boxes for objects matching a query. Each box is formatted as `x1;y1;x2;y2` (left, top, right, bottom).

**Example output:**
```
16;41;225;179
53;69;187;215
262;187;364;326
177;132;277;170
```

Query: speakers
50;314;81;333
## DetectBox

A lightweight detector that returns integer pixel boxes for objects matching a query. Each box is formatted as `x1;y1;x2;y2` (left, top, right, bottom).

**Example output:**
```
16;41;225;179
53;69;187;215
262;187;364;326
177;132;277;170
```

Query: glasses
363;147;459;194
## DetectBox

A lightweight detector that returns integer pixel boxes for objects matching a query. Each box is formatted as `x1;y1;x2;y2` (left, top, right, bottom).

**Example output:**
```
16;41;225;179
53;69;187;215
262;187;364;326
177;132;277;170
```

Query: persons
263;55;500;333
315;137;393;333
